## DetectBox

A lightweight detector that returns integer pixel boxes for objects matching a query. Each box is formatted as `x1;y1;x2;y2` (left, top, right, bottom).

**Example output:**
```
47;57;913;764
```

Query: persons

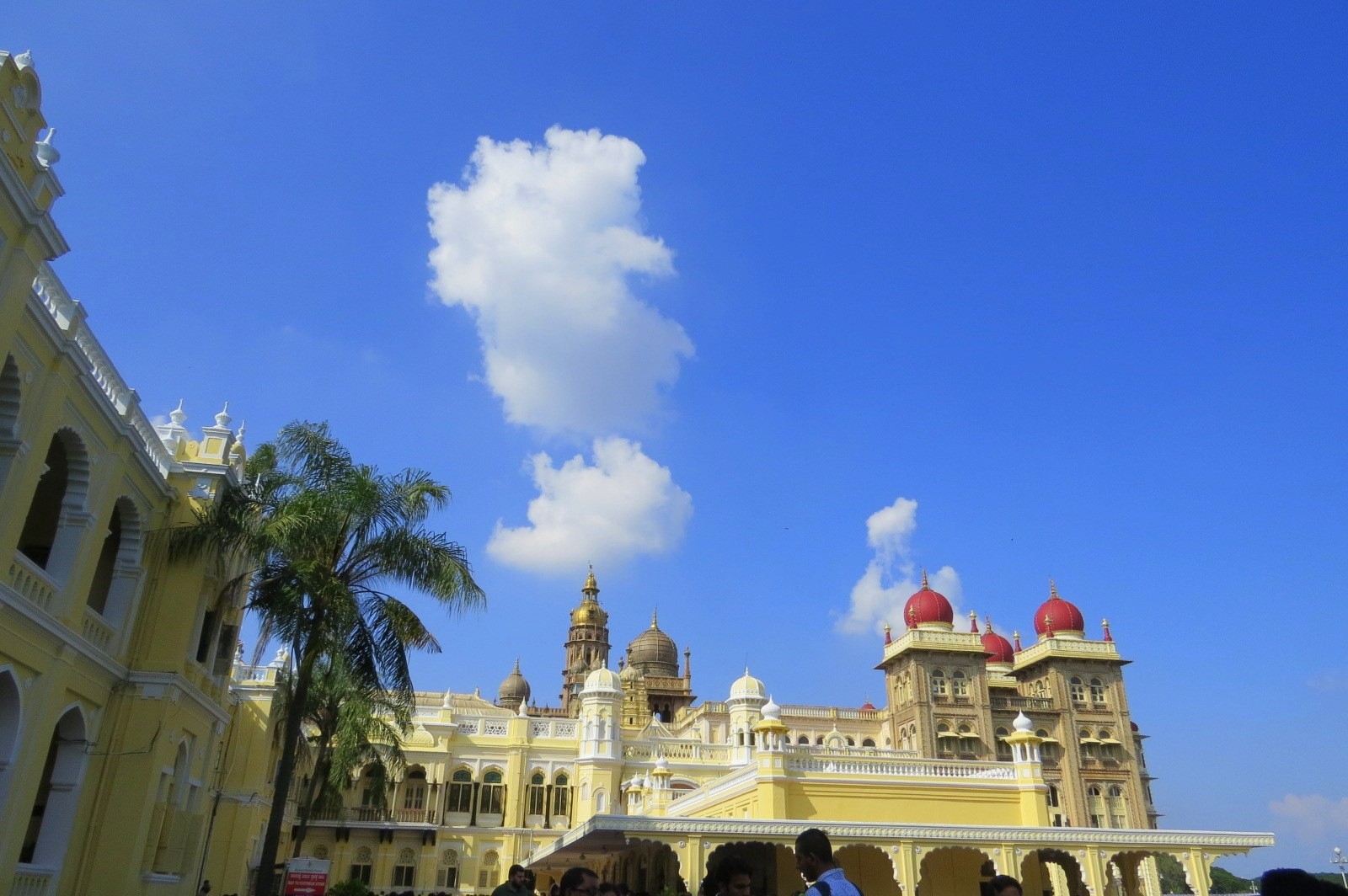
714;855;753;896
791;828;865;896
492;865;529;896
985;874;1022;896
560;864;652;896
